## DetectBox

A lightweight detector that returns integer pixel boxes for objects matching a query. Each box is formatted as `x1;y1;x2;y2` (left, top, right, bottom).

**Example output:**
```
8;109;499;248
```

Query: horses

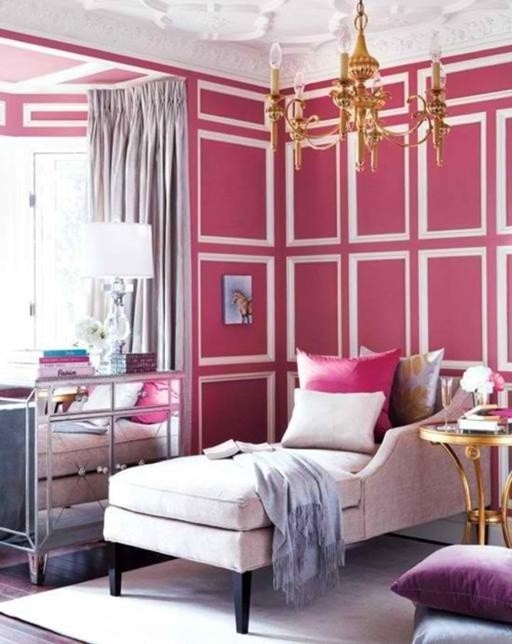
231;288;252;324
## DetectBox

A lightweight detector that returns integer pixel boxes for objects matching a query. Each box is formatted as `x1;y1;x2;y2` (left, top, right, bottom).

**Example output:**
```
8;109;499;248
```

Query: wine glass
438;375;454;433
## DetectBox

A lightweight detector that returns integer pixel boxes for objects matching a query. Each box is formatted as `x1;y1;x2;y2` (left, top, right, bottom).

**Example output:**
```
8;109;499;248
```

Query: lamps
72;222;153;354
266;0;448;173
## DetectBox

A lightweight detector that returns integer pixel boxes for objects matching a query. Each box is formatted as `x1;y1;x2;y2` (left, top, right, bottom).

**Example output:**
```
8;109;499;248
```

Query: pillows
279;343;459;453
390;543;511;644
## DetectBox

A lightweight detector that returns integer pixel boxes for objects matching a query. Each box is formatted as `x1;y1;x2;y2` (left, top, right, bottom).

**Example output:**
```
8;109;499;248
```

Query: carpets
0;537;440;642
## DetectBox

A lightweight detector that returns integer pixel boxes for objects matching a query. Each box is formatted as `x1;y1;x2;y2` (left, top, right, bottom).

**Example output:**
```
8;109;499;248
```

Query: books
35;349;96;380
202;438;275;460
109;351;158;374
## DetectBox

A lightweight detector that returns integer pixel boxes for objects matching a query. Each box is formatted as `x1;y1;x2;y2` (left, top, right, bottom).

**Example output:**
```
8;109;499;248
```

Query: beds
99;374;491;636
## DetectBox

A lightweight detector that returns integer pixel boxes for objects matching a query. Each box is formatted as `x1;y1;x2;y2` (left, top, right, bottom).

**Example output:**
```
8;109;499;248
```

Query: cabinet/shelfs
0;369;181;583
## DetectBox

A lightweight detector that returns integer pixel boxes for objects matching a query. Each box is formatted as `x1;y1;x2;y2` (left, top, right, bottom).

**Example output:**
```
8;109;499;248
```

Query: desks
416;421;512;545
